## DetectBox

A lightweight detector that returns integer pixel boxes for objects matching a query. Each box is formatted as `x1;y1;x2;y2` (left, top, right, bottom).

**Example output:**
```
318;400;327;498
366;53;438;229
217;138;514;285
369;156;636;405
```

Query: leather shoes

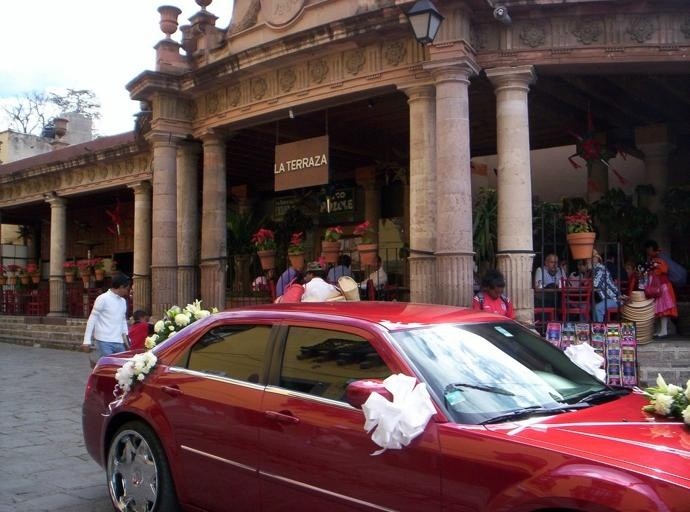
656;333;670;341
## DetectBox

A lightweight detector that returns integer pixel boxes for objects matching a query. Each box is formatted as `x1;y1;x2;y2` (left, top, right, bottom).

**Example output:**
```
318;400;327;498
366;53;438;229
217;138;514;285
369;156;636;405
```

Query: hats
620;291;657;345
592;248;603;264
305;261;322;273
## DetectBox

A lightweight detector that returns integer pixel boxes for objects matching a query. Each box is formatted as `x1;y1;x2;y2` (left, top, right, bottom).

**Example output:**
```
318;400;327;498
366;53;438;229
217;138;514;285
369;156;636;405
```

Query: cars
83;300;635;510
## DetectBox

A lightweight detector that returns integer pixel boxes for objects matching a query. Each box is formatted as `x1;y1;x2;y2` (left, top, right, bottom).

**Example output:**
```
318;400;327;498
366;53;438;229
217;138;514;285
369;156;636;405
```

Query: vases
321;241;341;267
0;269;106;286
289;254;305;271
357;245;379;272
566;234;597;262
257;249;277;271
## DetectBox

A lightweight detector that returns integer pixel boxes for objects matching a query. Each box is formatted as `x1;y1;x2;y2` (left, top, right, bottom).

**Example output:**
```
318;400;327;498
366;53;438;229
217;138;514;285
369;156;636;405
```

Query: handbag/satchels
657;254;688;285
638;264;650;290
645;275;663;298
278;276;304;303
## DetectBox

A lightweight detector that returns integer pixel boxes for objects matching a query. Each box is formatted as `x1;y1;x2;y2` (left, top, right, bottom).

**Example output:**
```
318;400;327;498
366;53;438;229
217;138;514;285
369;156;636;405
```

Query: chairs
532;273;637;335
267;278;409;305
0;288;133;319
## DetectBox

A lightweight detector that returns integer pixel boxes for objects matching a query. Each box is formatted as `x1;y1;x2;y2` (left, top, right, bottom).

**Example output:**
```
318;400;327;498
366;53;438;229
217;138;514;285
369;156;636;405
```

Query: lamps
406;1;446;47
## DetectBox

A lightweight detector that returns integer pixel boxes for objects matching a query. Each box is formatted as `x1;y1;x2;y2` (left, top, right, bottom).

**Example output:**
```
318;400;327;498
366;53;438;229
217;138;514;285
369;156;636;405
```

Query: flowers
321;226;344;244
143;298;219;348
250;228;277;250
0;258;106;276
351;221;376;247
287;232;307;253
101;348;160;418
566;209;595;234
628;372;690;430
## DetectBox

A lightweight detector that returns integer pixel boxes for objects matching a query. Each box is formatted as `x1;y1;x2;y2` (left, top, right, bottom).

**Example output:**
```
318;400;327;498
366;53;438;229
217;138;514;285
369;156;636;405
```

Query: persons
251;254;387;303
127;310;151;351
534;240;677;339
472;270;514;320
81;274;132;369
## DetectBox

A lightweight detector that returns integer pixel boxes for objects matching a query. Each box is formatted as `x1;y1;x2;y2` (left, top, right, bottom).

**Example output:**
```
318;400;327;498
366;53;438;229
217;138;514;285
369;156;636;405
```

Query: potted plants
225;207;277;293
